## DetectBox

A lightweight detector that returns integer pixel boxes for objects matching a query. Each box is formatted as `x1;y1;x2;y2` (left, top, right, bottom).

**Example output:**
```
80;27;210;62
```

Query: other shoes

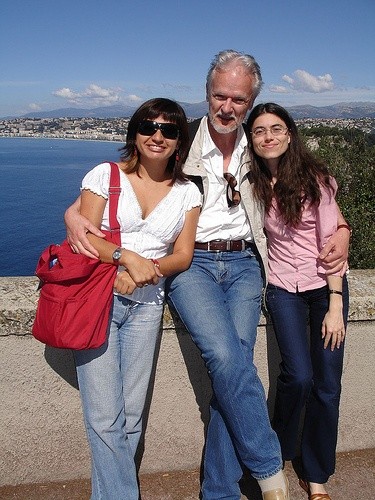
298;477;332;500
262;470;289;500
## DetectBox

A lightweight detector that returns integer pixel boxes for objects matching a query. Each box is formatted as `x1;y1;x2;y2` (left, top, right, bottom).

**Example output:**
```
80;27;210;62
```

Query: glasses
137;120;181;140
250;127;291;135
223;172;242;209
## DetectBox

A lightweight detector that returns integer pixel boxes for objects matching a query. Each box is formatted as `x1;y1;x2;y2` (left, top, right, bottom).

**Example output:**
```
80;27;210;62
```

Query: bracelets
329;289;343;295
151;257;160;271
337;224;352;238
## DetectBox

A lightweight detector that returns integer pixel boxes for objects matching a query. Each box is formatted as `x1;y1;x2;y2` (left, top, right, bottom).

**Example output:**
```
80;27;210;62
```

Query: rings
144;283;148;286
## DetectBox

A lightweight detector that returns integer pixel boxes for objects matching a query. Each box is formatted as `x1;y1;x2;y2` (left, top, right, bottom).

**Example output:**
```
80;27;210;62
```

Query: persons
75;98;202;500
238;103;348;500
63;49;352;500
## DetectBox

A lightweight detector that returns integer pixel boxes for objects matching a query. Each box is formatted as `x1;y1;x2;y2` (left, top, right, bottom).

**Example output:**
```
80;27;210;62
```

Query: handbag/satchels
30;161;122;350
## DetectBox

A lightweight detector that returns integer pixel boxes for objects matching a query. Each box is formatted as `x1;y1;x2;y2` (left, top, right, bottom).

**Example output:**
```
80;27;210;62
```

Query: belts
194;239;255;252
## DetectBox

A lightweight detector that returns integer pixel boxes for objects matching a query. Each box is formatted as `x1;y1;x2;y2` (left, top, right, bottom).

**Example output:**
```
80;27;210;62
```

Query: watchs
111;246;125;265
